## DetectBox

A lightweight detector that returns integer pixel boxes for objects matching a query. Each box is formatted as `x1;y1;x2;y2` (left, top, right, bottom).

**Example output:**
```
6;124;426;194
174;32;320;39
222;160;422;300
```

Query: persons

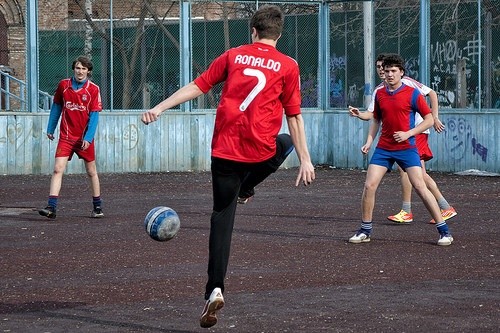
348;54;456;246
39;56;106;218
360;55;458;224
142;5;315;328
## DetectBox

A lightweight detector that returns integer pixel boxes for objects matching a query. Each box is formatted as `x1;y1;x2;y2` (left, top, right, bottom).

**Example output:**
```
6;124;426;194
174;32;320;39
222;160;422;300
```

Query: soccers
143;205;181;242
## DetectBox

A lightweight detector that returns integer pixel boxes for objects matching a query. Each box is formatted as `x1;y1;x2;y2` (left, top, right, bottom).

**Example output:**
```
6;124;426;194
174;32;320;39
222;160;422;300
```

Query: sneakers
437;231;454;245
91;206;104;217
429;205;458;223
348;230;370;243
237;195;253;204
38;206;57;219
387;210;413;222
200;287;225;328
